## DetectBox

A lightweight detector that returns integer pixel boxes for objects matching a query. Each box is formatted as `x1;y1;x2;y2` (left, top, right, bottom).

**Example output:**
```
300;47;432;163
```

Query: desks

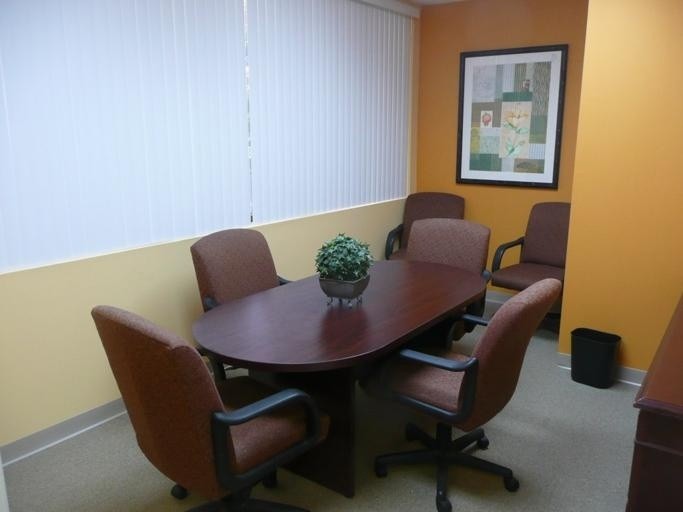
190;259;489;499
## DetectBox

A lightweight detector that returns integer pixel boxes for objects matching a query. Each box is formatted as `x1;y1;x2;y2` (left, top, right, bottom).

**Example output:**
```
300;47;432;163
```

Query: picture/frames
455;44;569;191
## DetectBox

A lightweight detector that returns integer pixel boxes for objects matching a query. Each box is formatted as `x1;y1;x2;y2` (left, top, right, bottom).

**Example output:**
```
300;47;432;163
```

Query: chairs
403;218;491;352
190;228;293;312
375;278;563;512
490;201;571;334
91;303;330;512
385;192;466;260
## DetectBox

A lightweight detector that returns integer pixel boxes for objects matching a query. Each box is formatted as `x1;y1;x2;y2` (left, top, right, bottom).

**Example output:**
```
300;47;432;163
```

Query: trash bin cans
570;328;621;388
